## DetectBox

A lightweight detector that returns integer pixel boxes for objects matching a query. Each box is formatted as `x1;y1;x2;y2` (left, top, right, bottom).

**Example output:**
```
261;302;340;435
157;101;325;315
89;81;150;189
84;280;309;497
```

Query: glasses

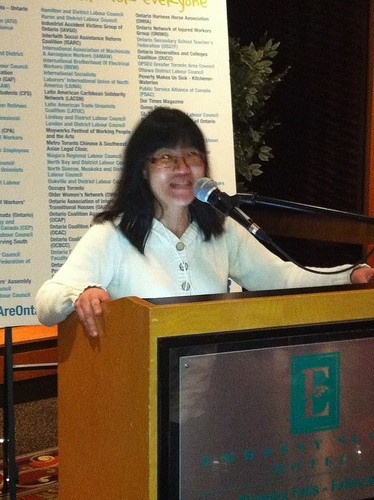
144;148;209;170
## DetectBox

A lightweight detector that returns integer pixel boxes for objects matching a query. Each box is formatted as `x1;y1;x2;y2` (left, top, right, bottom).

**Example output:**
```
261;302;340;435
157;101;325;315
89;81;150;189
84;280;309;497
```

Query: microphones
194;177;272;247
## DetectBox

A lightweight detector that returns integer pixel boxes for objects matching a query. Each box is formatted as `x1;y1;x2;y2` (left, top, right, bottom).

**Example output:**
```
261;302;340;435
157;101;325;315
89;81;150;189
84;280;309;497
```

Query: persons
32;106;373;342
